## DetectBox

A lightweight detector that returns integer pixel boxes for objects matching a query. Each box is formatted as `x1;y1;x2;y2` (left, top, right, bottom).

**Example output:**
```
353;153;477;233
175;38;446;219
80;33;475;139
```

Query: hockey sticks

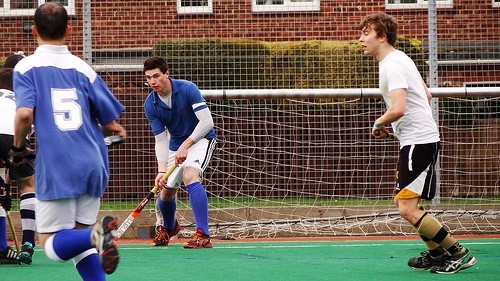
111;162;178;240
5;210;22;265
373;128;399;141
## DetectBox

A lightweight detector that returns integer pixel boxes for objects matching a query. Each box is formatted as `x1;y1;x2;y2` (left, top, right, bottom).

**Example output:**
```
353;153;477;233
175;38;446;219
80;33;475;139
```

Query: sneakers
407;251;449;270
151;219;181;246
183;228;213;248
435;249;477;275
0;246;20;264
17;242;34;265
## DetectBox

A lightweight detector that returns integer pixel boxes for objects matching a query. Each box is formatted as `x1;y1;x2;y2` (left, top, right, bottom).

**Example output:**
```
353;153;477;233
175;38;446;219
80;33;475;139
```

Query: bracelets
373;122;382;128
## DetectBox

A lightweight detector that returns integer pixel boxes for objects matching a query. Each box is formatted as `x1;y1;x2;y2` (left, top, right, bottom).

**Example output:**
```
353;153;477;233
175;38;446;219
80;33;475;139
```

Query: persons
0;51;35;265
359;11;477;275
7;2;127;281
143;56;217;249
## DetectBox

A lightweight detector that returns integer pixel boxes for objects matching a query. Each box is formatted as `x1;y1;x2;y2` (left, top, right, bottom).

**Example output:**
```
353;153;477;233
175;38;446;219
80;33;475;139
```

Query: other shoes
93;215;119;274
